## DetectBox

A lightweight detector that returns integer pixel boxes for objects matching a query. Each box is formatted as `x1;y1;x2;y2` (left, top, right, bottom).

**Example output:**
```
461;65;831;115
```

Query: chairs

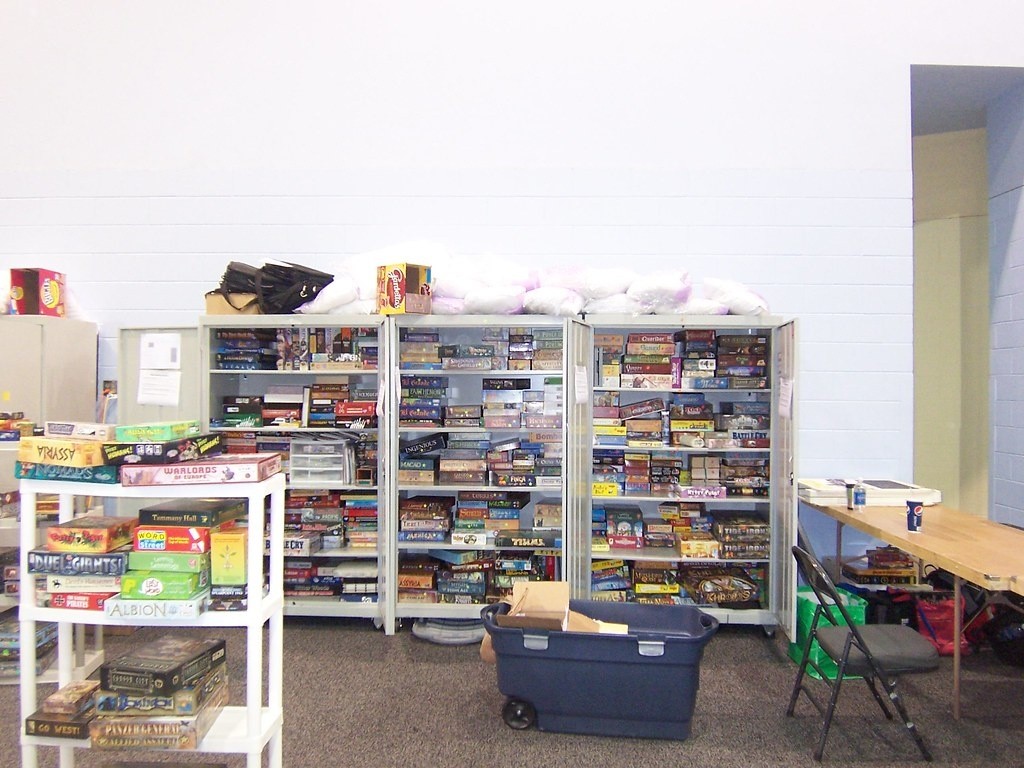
788;543;940;761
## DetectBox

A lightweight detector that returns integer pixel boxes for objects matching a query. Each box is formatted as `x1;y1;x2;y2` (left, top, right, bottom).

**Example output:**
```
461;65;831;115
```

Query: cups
846;484;855;510
906;499;923;532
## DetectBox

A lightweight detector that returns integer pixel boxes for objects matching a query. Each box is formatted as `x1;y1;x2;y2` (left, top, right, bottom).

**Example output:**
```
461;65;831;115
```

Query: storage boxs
376;261;432;317
0;323;767;768
9;266;65;316
842;562;917;584
860;589;956;648
204;288;263;315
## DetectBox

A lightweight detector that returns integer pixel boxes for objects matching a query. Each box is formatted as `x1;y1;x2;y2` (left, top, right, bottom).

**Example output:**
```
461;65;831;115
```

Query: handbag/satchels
916;588;973;656
204;260;334;314
786;585;869;680
924;564;1024;665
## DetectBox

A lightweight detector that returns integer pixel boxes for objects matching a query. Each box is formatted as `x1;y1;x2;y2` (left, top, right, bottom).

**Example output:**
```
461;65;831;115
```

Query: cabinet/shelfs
202;316;386;631
0;443;105;685
14;474;285;768
569;314;799;644
389;317;568;632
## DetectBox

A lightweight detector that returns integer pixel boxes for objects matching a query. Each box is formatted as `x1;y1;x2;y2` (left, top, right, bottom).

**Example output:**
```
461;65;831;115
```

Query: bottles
854;476;867;514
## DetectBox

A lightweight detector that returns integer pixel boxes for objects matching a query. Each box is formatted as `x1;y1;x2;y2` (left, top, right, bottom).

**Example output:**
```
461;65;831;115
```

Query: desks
799;493;1023;719
